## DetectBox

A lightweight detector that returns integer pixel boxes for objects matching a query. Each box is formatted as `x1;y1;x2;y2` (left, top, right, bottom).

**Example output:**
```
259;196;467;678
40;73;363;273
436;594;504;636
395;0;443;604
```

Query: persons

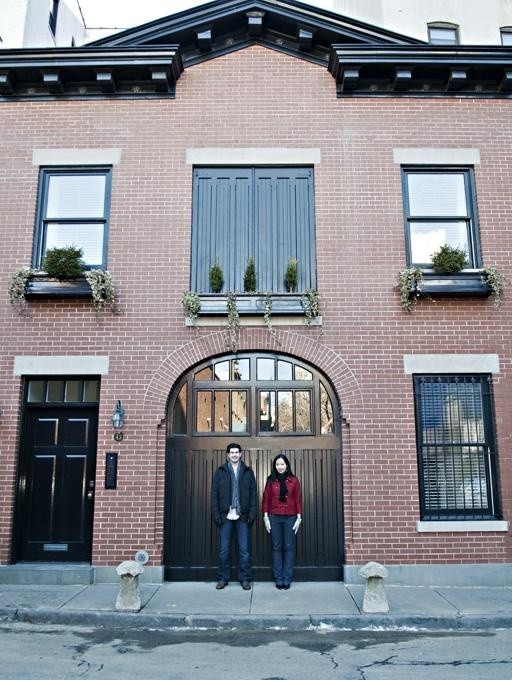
210;443;258;589
262;454;303;590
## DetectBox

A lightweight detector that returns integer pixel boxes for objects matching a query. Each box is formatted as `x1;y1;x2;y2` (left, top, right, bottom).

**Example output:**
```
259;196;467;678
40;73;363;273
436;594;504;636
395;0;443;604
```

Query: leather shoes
276;583;290;590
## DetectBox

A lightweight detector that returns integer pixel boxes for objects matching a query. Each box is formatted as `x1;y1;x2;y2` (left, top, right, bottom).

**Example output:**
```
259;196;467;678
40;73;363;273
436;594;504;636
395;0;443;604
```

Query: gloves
214;516;224;526
247;516;254;527
292;516;303;536
263;516;272;534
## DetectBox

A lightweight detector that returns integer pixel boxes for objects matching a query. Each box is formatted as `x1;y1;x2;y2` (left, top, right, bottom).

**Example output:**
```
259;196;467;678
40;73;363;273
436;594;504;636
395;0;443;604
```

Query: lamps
110;400;125;442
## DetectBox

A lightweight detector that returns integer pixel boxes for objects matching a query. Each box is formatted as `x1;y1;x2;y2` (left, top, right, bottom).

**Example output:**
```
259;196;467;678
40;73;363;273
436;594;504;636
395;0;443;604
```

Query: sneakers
216;581;228;589
240;581;251;590
